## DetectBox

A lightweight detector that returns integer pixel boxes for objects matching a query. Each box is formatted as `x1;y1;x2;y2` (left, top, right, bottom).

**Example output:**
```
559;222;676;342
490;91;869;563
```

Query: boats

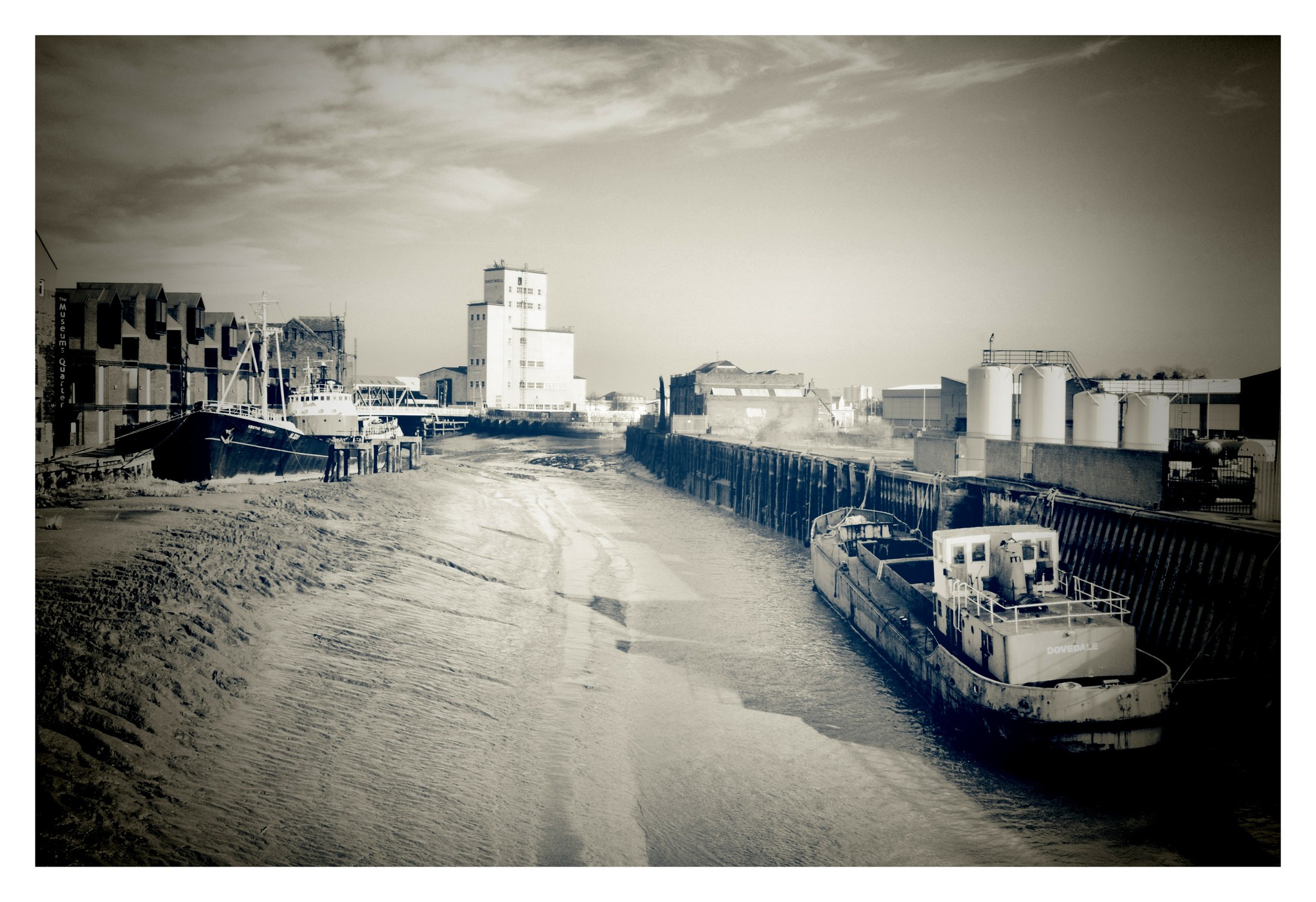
112;287;405;484
802;505;1176;767
